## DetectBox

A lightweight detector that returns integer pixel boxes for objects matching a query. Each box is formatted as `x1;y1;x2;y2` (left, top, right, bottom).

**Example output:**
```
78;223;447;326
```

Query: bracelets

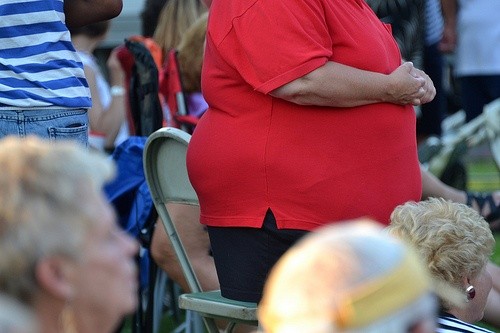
110;85;127;96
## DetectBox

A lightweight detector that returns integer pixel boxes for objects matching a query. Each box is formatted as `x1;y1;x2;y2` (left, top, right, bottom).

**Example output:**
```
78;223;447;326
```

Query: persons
257;215;437;332
151;10;500;333
359;0;500;143
0;135;140;333
387;196;500;332
59;0;211;156
186;0;436;304
0;0;124;145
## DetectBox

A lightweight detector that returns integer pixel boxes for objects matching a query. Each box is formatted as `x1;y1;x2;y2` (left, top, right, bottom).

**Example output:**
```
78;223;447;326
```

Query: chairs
142;127;258;333
